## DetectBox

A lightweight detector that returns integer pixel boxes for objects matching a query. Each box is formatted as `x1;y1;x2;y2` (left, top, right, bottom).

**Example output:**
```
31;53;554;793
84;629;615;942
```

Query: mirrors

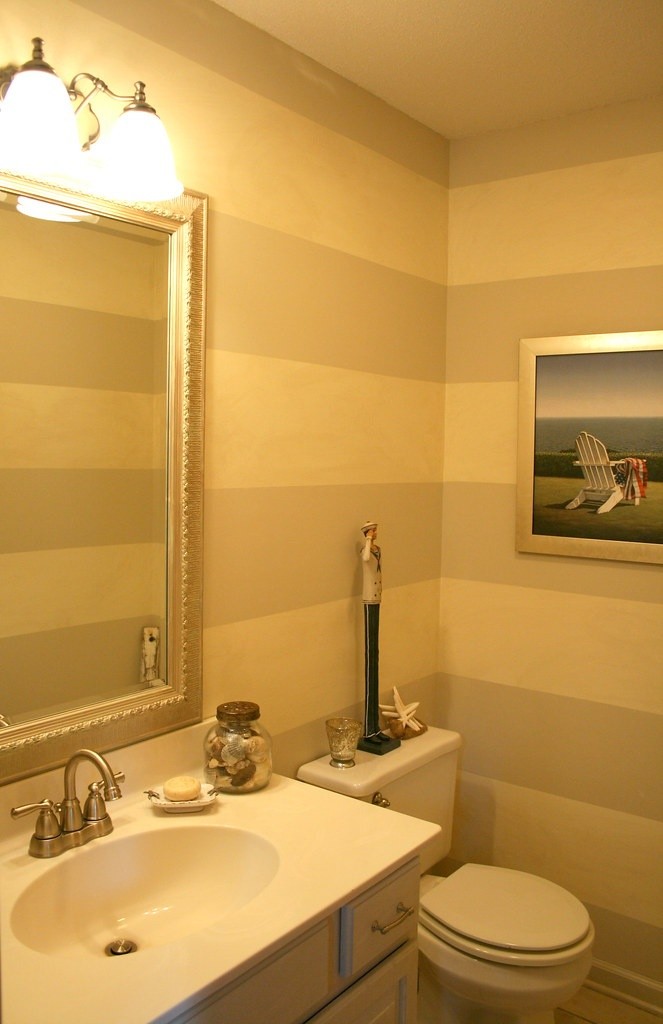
0;170;209;786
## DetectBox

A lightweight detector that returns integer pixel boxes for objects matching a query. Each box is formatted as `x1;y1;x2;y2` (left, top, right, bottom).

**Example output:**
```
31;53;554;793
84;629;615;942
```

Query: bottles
203;702;273;794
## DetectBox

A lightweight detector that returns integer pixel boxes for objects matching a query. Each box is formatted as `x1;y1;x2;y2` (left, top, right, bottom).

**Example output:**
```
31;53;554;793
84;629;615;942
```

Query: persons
359;521;390;746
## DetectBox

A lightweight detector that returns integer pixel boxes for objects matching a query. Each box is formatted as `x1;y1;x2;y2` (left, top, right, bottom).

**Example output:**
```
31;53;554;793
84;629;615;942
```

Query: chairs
564;431;647;514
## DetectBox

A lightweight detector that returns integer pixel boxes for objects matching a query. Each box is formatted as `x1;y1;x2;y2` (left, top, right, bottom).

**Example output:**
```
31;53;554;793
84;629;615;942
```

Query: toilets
297;724;596;1024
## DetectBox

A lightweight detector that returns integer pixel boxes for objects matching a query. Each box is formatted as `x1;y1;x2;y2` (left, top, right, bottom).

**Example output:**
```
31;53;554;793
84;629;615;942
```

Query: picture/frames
514;330;663;565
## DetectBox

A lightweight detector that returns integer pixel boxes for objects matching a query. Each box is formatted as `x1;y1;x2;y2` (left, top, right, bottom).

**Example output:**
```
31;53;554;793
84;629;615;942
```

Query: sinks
10;826;280;967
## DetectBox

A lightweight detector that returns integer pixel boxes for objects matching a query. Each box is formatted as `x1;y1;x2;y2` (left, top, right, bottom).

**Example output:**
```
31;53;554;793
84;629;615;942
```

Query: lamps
0;38;186;205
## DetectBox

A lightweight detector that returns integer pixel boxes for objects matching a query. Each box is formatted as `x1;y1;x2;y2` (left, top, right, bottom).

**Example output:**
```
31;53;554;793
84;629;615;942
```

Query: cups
326;718;361;767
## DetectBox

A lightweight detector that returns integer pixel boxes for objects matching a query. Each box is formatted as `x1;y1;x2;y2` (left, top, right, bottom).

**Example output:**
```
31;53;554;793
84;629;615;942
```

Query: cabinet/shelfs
170;857;422;1024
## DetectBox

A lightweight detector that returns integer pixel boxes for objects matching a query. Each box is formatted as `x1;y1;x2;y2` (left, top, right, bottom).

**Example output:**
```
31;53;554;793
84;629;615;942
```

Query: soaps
164;775;200;802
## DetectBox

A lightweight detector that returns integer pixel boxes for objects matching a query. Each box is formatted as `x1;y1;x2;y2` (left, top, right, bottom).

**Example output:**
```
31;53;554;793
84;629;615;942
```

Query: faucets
63;750;121;834
0;714;10;726
